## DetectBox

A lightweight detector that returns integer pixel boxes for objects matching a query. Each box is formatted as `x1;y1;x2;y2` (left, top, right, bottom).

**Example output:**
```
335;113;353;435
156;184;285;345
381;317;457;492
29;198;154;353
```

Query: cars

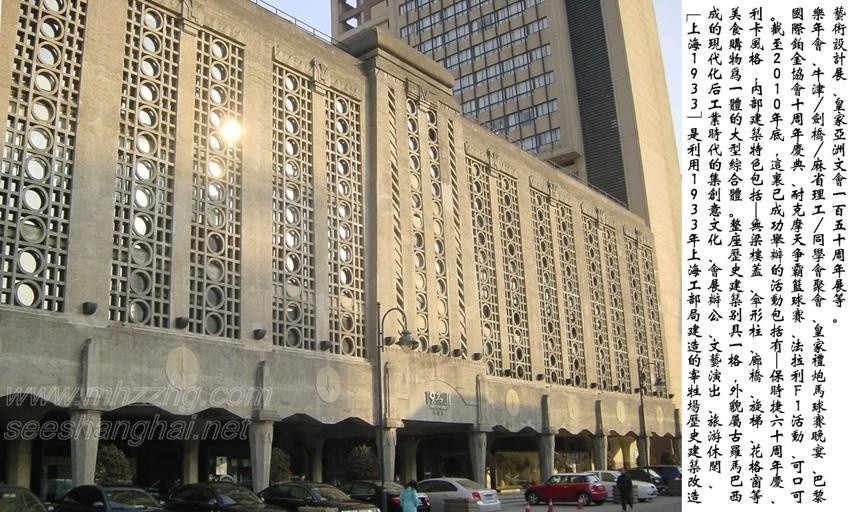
524;466;682;506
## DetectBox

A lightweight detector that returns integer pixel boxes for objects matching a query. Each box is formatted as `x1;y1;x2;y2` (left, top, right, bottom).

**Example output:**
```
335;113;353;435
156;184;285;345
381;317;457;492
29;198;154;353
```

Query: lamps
378;308;419;353
639;362;667;387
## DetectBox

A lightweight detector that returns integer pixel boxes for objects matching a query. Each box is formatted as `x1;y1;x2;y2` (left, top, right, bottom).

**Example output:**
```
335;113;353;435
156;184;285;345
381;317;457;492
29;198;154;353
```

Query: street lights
378;307;418;512
638;361;663;480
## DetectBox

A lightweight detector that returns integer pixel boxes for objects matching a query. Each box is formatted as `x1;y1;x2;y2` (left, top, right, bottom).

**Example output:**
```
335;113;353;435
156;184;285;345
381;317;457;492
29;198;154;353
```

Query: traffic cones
525;495;587;511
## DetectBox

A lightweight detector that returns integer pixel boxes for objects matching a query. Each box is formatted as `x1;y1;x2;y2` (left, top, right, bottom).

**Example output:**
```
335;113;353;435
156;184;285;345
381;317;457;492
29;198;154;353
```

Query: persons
616;467;634;511
399;479;419;512
